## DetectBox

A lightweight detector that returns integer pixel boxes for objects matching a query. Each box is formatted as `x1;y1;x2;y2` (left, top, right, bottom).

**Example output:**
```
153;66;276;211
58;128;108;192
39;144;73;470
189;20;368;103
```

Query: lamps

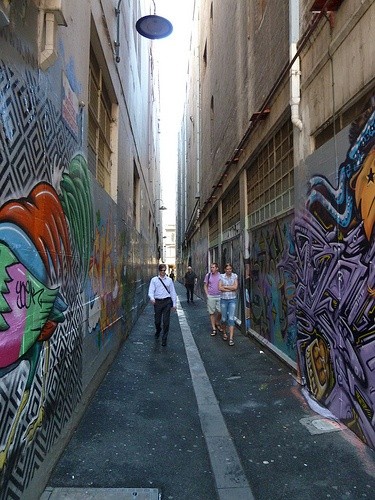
154;199;167;210
113;0;173;63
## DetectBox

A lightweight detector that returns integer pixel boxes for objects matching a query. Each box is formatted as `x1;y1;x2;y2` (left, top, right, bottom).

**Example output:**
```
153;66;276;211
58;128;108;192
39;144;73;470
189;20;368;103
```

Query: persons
219;263;239;347
204;263;226;336
185;266;197;305
149;264;177;348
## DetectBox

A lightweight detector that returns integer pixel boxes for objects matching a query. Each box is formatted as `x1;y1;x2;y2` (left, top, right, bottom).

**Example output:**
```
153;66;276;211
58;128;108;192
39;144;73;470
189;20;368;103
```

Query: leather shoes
162;340;167;346
155;328;162;338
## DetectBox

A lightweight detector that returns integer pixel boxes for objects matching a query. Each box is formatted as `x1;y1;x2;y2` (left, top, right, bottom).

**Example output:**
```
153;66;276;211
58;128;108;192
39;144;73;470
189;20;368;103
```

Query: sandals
228;338;235;346
216;325;223;332
210;330;217;336
223;335;228;340
